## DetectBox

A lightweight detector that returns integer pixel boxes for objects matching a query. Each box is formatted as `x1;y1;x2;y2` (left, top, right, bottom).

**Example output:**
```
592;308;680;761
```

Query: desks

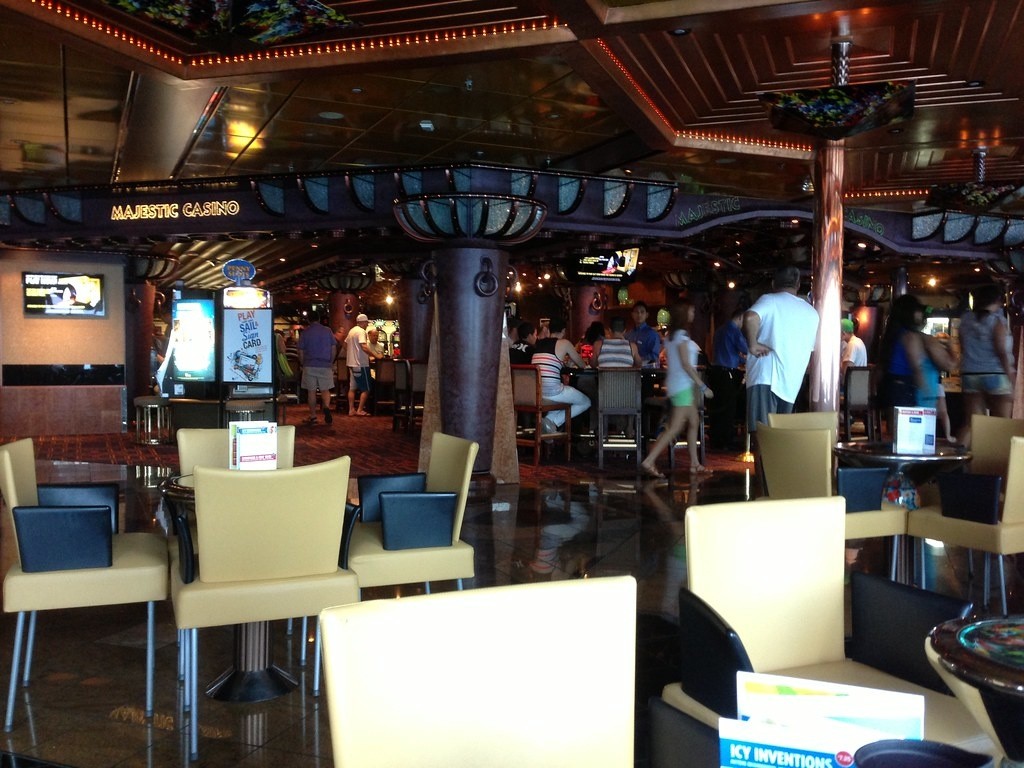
835;438;971;582
157;470;298;705
926;613;1024;768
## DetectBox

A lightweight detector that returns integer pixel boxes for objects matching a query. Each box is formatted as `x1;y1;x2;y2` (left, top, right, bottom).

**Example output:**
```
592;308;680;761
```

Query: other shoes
541;418;559;444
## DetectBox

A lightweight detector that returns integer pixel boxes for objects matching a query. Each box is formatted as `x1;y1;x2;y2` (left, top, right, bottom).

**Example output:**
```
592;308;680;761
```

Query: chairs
0;355;1023;767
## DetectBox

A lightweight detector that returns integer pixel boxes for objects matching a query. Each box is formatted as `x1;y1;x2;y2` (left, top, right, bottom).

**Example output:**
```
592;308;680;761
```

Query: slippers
356;412;371;417
349;414;356;417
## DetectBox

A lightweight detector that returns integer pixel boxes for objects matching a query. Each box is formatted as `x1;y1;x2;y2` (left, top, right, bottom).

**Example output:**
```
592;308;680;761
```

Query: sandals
323;407;332;423
690;463;713;474
304;417;317;423
642;463;665;478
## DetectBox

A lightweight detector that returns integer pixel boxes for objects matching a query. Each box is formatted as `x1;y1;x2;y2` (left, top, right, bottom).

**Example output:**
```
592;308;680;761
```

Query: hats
841;319;854;333
356;314;372;323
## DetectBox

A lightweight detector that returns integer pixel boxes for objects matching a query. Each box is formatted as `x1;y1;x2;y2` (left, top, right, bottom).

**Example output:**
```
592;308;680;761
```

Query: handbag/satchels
276;334;294;377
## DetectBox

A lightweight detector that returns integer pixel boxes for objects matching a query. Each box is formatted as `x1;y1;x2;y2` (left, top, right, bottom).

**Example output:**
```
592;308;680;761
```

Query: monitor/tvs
21;271;109;320
569;244;640;285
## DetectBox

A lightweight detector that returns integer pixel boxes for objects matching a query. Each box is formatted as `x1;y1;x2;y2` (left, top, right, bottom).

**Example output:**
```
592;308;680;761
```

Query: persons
877;279;1024;450
641;264;820;479
274;329;294;394
839;317;868;393
343;314;385;416
506;299;661;456
298;311;337;426
